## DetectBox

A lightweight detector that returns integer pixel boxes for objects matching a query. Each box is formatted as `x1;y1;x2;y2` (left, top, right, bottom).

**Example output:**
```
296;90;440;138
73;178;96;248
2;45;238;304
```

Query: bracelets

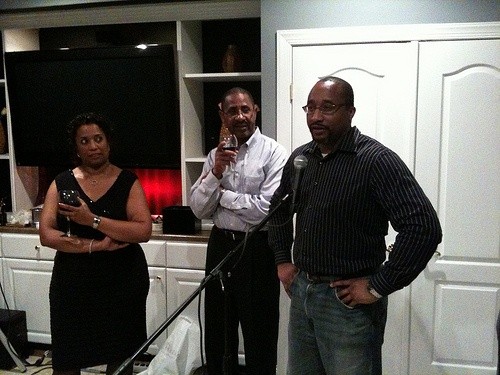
88;238;95;254
366;276;385;300
92;214;102;229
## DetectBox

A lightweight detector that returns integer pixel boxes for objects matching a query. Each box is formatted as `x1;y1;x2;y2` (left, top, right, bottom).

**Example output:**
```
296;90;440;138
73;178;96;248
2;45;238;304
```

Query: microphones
288;155;308;217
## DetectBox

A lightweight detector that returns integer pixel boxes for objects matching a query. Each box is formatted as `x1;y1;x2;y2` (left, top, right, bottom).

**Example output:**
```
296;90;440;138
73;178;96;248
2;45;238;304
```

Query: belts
214;225;268;240
299;270;372;282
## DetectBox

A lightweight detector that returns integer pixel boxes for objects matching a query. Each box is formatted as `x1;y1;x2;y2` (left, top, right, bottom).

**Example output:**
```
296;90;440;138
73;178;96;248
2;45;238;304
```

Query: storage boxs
163;206;201;234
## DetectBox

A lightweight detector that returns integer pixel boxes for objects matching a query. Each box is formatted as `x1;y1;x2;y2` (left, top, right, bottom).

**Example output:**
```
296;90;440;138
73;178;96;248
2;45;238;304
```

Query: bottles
223;45;237;72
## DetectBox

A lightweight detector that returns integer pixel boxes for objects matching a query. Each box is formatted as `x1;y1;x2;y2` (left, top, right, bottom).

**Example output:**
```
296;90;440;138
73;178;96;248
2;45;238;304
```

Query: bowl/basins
32;208;43;223
34;221;39;229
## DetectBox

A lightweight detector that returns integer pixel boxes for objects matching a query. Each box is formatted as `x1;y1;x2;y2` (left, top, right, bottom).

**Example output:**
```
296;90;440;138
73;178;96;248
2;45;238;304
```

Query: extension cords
132;361;150;373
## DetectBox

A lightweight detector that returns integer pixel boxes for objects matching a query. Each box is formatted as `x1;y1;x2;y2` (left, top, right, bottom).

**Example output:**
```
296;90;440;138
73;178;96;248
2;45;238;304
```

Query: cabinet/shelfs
0;0;261;366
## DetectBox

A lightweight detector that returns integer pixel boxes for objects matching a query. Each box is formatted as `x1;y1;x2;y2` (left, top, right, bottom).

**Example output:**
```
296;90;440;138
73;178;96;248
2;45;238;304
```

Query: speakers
162;206;202;234
0;308;29;371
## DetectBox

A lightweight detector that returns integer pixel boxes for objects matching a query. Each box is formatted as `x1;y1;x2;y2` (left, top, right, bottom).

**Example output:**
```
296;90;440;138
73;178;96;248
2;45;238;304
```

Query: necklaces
81;164;109;185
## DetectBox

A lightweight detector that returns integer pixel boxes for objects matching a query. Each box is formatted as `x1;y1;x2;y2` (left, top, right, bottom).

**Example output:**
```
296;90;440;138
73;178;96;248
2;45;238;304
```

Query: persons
40;113;152;375
268;76;443;375
188;87;290;375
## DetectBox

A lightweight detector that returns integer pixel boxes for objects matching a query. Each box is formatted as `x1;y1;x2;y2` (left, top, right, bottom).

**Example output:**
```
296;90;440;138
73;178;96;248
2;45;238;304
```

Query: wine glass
221;134;238;174
60;190;79;237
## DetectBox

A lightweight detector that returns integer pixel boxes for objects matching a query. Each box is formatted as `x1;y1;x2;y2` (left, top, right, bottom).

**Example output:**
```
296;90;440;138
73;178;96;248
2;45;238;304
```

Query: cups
151;221;162;231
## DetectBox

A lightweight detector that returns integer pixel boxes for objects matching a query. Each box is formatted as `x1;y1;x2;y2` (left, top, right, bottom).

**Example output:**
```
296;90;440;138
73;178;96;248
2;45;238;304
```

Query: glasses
222;105;254;118
302;103;349;115
